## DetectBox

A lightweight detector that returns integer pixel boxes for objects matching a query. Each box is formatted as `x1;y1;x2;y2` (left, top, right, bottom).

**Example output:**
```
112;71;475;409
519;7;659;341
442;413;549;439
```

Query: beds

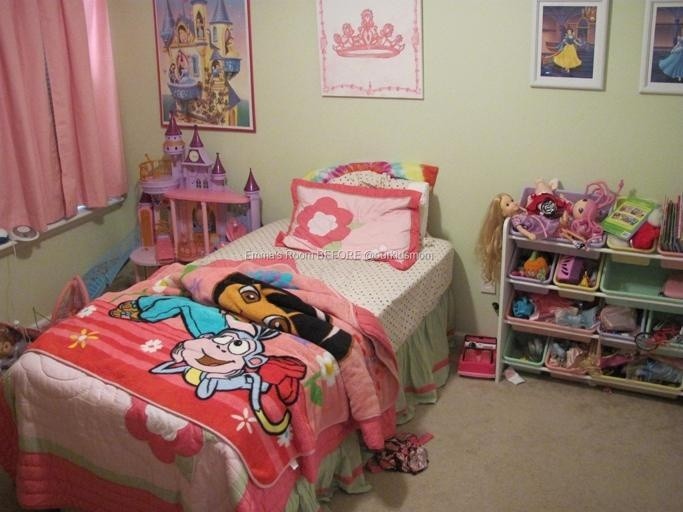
0;221;454;512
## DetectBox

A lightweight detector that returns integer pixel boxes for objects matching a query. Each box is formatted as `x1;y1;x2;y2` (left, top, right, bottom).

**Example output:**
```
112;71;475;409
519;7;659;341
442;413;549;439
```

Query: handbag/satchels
601;304;639;333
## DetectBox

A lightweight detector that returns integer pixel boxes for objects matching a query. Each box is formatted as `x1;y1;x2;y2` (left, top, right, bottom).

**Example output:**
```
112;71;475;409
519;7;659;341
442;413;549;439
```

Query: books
599;193;656;242
659;190;682;254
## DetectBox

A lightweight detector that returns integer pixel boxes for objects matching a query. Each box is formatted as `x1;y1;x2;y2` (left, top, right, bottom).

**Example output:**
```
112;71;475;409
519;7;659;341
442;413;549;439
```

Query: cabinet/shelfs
492;216;683;399
129;189;249;283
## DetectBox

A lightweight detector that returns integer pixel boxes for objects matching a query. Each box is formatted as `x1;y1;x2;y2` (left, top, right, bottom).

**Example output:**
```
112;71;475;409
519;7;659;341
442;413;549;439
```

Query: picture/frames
529;0;608;91
638;0;683;94
153;0;256;133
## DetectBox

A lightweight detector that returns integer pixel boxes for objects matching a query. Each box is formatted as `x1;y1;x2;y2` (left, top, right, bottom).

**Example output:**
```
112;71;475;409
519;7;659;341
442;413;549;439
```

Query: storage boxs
503;186;683;393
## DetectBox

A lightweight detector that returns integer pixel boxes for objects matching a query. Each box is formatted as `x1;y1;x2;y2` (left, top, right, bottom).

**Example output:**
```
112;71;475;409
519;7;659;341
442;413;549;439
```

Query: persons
520;174;625;250
473;192;583;295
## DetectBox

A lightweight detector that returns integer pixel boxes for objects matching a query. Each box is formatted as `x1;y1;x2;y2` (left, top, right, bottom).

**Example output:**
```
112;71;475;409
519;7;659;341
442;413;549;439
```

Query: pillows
275;178;422;270
326;170;430;253
298;161;438;194
